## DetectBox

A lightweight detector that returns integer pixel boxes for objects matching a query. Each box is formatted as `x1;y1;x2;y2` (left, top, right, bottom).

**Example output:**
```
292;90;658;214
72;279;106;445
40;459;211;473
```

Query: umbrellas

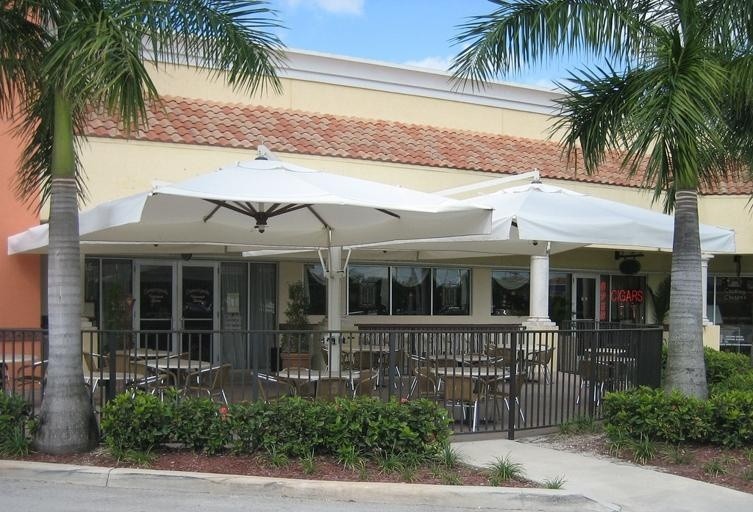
241;170;736;372
7;141;492;372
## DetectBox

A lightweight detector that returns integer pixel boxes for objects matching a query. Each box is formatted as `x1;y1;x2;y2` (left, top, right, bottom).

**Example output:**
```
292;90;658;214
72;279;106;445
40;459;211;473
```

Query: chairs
1;345;232;414
707;305;741;353
250;345;556;433
575;344;633;410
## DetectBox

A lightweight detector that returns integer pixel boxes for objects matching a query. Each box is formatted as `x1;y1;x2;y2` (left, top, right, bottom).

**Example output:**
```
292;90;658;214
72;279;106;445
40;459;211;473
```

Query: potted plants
280;280;315;369
